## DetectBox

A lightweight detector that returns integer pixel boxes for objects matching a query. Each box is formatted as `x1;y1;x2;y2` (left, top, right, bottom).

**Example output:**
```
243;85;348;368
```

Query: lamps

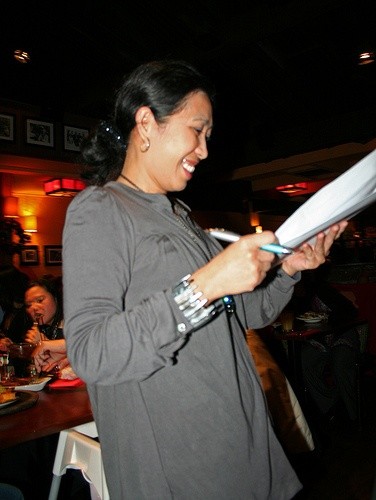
3;186;37;233
44;176;87;197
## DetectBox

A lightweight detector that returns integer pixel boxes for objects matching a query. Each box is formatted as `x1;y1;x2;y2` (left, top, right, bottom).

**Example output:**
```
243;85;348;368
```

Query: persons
293;277;366;432
0;220;70;369
61;58;348;500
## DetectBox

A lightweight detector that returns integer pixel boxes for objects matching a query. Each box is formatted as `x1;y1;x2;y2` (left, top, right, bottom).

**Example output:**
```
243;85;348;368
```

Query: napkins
48;378;83;391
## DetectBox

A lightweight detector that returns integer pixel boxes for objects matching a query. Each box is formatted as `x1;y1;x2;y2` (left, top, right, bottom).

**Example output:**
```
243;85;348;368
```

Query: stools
320;352;364;435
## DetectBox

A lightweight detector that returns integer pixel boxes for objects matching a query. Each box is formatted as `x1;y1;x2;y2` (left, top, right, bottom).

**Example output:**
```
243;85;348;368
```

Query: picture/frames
0;111;17;146
62;122;91;154
19;244;39;266
44;244;62;266
24;116;57;151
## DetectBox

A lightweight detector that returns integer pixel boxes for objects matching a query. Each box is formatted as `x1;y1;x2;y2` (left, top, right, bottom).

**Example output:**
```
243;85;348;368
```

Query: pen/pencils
207;228;294;253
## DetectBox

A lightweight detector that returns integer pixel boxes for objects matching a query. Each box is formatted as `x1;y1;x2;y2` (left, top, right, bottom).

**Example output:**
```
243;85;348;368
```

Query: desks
266;319;369;457
0;379;94;449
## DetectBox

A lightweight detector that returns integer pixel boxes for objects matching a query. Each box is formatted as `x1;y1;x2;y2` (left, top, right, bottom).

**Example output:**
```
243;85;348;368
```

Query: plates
0;390;39;415
297;318;323;323
14;377;52;392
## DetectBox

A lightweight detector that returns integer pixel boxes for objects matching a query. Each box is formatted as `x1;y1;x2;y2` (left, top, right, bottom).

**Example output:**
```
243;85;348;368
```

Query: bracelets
171;274;217;329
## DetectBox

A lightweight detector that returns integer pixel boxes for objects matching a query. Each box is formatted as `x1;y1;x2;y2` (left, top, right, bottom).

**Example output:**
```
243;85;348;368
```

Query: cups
7;343;33;378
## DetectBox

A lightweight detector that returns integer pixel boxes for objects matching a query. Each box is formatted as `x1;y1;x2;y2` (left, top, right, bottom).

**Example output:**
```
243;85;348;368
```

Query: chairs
49;420;111;500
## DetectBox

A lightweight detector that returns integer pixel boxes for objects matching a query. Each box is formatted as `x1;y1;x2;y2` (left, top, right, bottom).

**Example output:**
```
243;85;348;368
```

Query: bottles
33;318;39;328
0;354;8;381
